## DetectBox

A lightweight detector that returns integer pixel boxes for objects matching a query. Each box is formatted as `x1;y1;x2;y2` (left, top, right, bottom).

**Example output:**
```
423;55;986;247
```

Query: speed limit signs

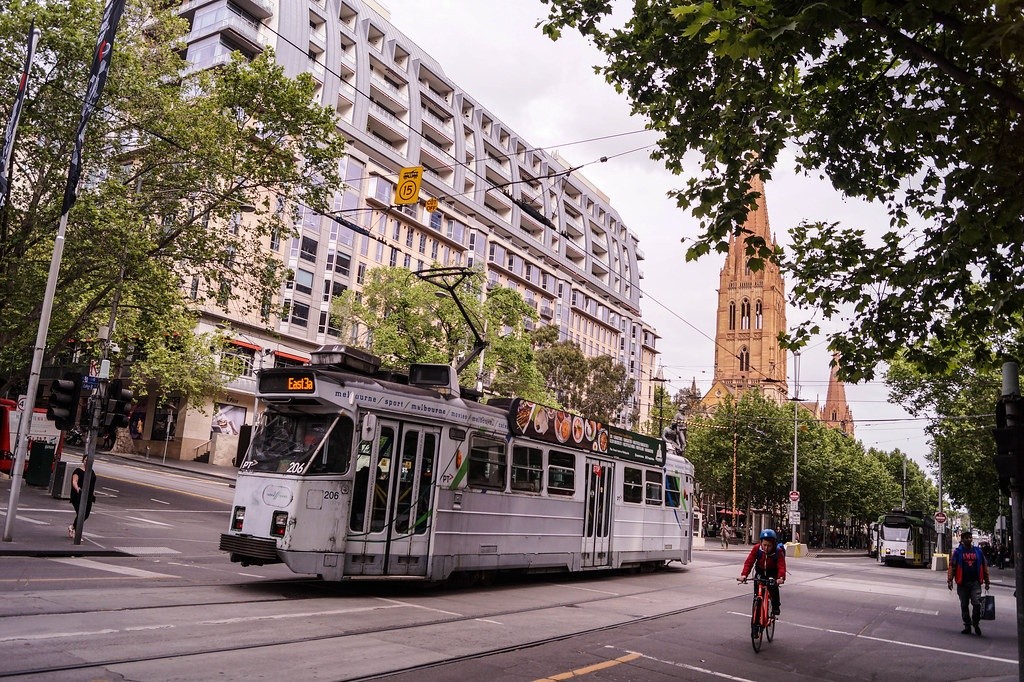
16;395;27;412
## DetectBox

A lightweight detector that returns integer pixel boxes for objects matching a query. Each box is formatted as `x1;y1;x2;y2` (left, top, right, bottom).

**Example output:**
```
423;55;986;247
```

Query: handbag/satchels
725;530;730;538
980;588;995;620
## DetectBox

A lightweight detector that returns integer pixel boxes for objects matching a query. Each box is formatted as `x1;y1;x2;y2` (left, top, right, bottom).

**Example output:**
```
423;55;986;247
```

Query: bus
218;345;694;587
869;510;953;569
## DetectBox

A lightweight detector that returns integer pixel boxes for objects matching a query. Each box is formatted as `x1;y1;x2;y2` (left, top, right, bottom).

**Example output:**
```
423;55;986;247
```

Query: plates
534;412;549;434
584;420;596;441
548;408;555;421
598;427;608;454
555;414;571;443
573;416;585;444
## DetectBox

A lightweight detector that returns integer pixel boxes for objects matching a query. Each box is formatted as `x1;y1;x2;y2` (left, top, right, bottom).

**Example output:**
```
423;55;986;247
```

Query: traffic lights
46;371;82;430
103;377;132;429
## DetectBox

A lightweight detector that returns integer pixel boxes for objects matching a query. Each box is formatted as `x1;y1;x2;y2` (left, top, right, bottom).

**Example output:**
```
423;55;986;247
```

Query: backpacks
755;542;786;574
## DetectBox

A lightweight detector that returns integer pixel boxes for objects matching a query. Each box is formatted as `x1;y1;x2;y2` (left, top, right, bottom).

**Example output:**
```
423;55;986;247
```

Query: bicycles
736;577;783;654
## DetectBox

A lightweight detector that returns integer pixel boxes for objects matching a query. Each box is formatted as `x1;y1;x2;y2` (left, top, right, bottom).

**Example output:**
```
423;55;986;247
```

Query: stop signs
789;490;801;502
934;513;947;523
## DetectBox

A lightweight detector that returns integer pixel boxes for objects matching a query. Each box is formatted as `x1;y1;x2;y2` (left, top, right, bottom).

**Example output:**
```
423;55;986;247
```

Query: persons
775;526;783;544
946;531;990;636
663;403;687;454
69;454;97;543
738;528;786;637
720;519;730;550
101;430;117;451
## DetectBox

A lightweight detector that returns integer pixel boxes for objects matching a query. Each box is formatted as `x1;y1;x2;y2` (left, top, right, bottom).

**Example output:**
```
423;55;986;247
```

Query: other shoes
69;525;74;538
751;628;758;638
80;538;84;542
772;607;781;614
971;622;981;636
961;628;972;634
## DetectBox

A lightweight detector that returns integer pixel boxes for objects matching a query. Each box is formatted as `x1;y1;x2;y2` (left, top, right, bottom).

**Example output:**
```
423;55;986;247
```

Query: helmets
759;529;776;539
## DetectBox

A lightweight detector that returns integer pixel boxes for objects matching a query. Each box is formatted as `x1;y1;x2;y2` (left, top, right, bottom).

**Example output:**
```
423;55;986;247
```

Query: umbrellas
717;508;744;515
